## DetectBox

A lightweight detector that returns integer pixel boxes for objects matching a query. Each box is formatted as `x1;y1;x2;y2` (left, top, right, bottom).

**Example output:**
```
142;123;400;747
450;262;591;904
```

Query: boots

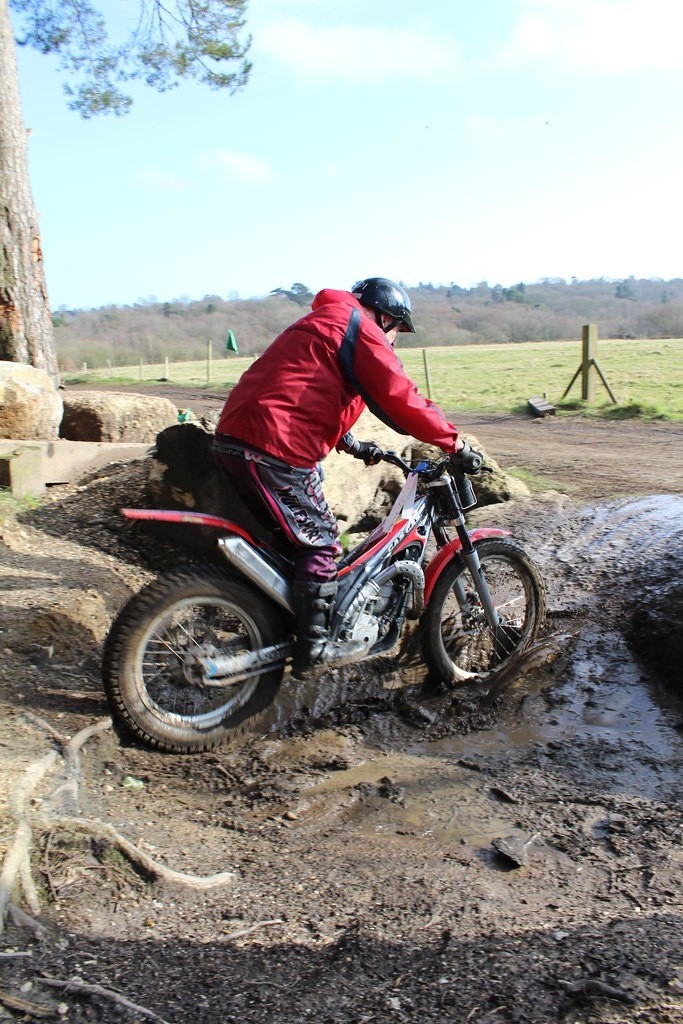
290;582;370;681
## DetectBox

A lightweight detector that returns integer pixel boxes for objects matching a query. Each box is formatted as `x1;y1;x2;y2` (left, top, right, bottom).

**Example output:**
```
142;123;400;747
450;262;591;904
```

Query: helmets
351;278;416;335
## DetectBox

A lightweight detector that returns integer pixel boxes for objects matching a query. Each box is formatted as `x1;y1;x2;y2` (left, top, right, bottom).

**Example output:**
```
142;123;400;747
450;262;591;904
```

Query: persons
211;276;486;682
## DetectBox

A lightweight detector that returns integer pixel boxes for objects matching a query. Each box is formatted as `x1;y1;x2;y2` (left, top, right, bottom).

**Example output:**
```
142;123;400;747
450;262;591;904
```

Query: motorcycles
99;425;544;758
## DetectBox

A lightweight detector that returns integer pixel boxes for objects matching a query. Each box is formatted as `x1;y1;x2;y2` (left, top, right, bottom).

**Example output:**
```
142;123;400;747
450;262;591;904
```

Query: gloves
457;441;484;474
354;441;383;466
